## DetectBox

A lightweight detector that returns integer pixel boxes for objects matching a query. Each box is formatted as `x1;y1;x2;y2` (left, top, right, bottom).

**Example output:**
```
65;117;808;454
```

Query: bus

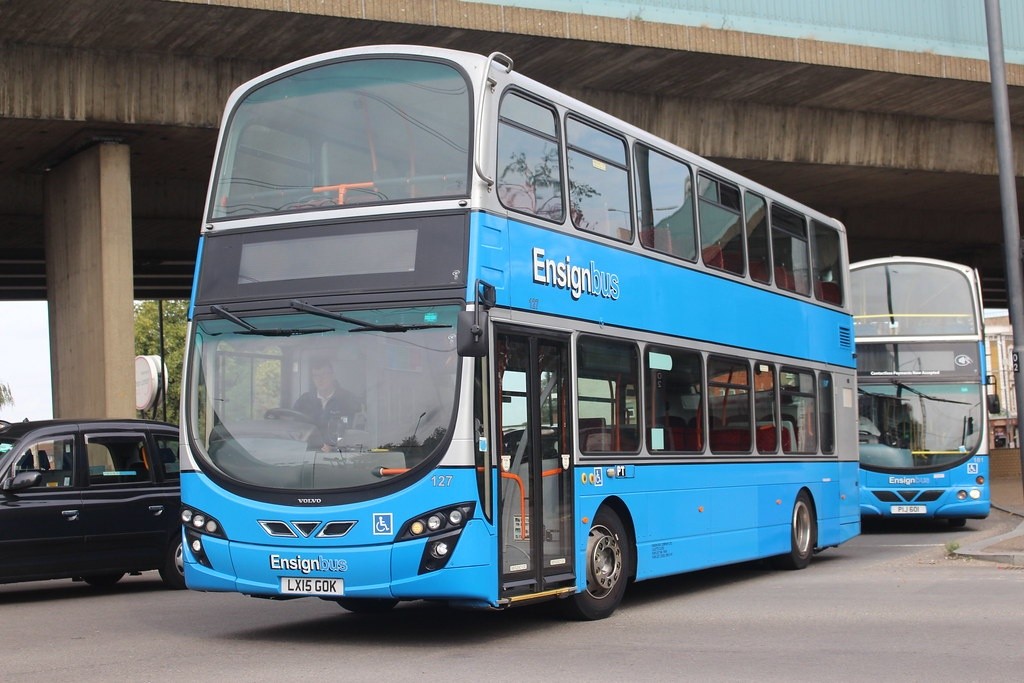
180;44;860;623
798;254;1000;529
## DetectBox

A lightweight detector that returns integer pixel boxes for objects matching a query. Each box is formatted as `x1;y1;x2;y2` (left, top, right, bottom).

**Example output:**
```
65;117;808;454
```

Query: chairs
578;418;637;451
124;444;139;473
497;152;843;306
160;448;176;463
646;414;797;451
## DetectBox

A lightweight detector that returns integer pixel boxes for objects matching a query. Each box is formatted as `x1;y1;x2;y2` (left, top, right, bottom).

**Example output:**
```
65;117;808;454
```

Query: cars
0;418;188;592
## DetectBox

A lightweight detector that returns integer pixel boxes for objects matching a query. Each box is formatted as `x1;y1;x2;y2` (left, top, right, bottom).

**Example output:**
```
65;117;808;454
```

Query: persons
292;356;367;453
858;398;881;436
